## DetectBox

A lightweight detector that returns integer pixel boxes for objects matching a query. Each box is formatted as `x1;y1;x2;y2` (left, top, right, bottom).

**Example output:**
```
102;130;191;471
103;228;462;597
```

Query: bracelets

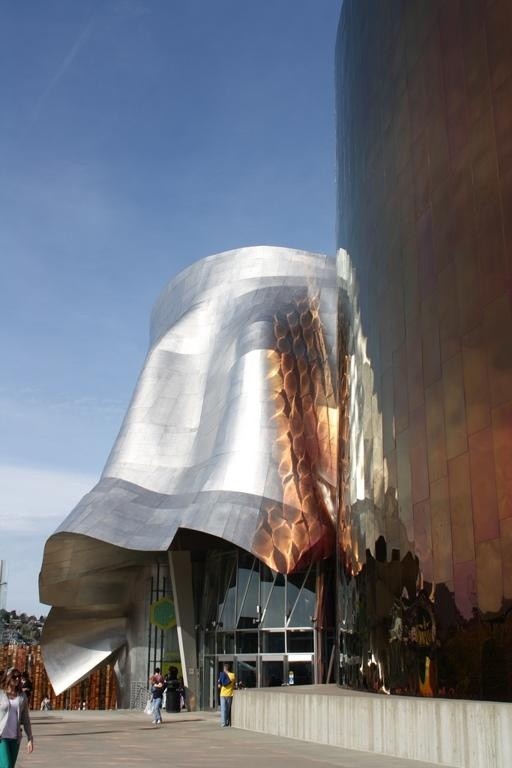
29;736;32;740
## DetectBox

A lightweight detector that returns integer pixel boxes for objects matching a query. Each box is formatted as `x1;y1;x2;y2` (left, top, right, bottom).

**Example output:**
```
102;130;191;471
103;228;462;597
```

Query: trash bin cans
165;680;180;713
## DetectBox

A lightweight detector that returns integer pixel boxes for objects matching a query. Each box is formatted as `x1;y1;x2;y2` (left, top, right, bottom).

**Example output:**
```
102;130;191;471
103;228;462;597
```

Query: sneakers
152;720;162;723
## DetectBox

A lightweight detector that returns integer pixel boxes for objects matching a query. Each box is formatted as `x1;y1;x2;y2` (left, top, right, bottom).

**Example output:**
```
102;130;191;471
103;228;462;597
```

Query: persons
150;667;168;723
218;664;236;727
20;671;33;706
0;667;33;768
41;694;51;712
